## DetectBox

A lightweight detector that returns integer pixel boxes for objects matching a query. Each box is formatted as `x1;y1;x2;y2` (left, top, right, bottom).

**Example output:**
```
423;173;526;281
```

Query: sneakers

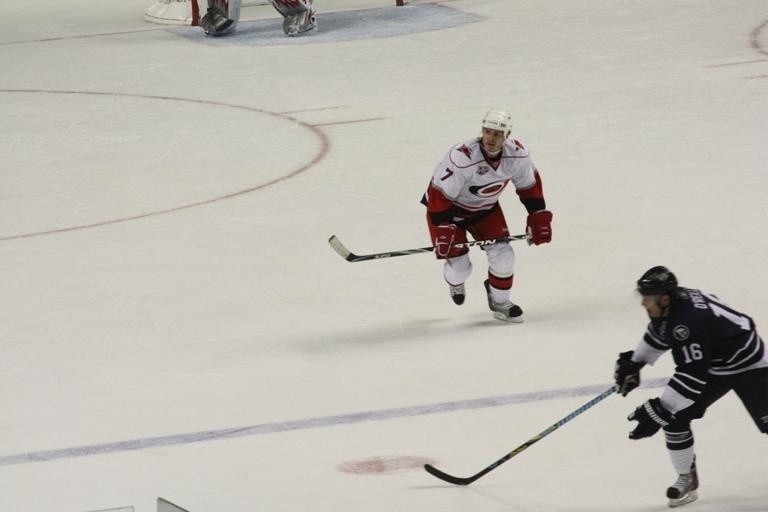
667;453;699;499
488;298;523;317
199;4;240;35
449;281;465;306
283;0;317;35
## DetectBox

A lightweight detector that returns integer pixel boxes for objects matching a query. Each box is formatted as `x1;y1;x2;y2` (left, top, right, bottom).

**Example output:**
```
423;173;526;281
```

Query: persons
614;266;768;506
419;106;552;323
198;0;317;37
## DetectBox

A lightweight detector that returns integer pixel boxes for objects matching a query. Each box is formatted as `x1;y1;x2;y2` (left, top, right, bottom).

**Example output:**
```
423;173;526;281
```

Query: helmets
481;105;514;142
637;266;678;303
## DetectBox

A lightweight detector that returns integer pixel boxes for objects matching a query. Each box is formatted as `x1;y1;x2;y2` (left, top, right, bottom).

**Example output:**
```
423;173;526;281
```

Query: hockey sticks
328;234;532;262
424;385;621;486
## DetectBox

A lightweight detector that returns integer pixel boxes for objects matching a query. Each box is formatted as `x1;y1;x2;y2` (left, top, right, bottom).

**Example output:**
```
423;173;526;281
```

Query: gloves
434;223;458;259
627;397;676;441
614;350;647;397
525;209;554;247
